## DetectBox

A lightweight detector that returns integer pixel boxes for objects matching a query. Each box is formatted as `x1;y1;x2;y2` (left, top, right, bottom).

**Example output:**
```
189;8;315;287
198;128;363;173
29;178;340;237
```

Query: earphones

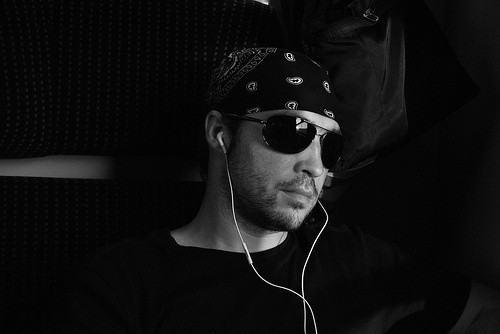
217;131;227;154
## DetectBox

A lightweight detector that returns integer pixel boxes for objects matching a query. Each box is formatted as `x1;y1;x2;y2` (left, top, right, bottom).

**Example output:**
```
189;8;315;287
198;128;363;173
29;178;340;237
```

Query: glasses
224;113;347;171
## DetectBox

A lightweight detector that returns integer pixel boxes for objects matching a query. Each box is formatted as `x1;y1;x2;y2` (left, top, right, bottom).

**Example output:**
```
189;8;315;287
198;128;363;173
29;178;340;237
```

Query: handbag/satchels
303;0;409;170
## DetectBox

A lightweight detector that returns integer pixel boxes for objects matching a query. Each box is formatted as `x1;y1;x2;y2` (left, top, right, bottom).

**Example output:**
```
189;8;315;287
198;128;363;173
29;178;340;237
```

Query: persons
51;48;436;334
